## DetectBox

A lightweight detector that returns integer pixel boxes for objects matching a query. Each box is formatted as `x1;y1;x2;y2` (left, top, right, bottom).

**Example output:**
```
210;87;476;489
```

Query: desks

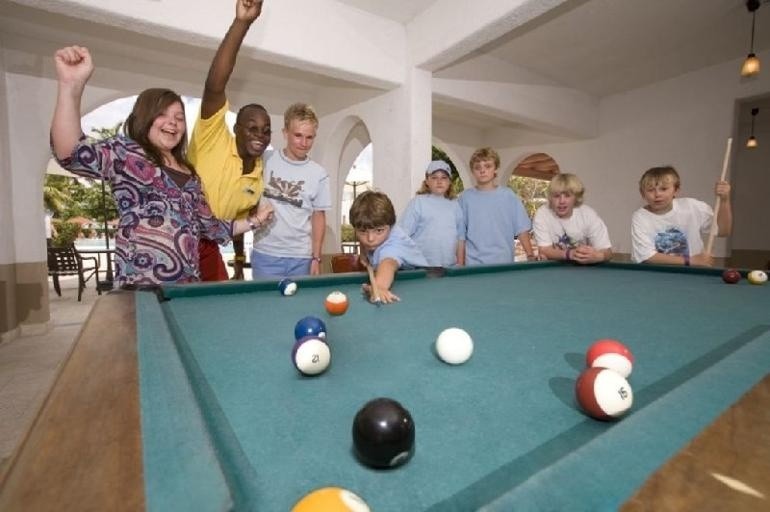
0;256;770;512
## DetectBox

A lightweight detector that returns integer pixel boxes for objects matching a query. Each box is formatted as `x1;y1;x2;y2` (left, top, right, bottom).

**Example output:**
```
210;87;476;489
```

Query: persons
398;160;467;268
630;166;734;267
250;103;334;278
532;173;614;265
456;146;549;265
349;190;431;305
185;0;270;281
48;44;273;290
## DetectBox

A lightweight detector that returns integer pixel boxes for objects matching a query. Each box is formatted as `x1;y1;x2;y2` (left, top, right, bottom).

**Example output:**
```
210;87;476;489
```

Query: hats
426;160;452;179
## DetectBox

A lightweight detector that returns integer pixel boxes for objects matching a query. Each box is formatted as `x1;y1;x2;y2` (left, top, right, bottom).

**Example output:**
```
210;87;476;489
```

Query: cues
367;263;382;307
707;138;734;253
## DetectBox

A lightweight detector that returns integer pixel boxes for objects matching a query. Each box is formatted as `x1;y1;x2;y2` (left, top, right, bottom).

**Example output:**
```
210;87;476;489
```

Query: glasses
237;123;273;137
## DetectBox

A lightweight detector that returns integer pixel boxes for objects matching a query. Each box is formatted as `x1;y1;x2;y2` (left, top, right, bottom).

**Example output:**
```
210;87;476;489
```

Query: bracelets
313;256;321;261
246;213;265;230
566;248;571;260
527;255;535;258
684;254;691;266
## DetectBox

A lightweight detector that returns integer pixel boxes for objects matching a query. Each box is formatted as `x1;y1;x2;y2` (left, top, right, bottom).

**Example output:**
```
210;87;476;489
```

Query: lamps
740;0;760;78
746;107;760;150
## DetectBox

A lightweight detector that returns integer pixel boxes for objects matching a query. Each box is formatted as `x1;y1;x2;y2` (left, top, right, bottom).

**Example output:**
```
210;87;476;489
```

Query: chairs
47;237;99;301
331;253;366;274
